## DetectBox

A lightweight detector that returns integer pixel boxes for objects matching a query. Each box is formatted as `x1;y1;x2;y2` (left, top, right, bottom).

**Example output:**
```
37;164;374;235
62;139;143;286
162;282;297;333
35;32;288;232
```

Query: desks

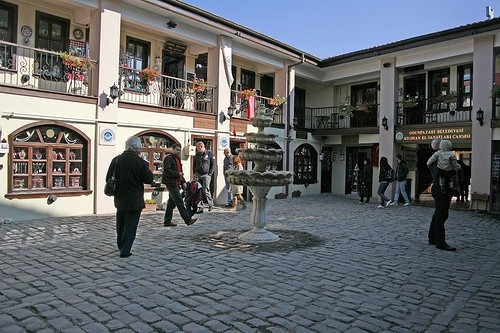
315;116;330;128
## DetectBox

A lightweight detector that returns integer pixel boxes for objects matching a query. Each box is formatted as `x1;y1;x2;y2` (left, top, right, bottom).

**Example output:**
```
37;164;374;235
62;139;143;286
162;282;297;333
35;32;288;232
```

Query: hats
395;154;402;161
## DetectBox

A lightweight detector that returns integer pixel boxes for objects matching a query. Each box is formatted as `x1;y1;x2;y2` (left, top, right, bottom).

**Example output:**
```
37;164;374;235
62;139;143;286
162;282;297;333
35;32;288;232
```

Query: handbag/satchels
104;176;117;196
359;184;368;197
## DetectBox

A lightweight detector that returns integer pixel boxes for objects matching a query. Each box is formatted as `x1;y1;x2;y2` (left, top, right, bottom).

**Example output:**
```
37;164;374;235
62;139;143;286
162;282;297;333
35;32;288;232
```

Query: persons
376;157;392;208
106;136;153;258
457;160;471;201
163;144;197;226
229;157;248;211
426;140;461;193
194;141;216;214
223;148;235;207
426;138;457;251
390;154;411;206
184;172;202;217
358;158;373;205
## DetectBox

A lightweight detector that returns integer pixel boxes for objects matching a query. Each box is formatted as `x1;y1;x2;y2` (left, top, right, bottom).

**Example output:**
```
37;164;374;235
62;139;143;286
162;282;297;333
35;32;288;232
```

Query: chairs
327;112;339;128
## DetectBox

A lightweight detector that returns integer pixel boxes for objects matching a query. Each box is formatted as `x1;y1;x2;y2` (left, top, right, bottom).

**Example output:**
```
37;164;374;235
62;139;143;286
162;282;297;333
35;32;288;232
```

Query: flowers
139;67;157;81
239;89;256;100
61;50;94;70
191;78;207;92
268;95;285;106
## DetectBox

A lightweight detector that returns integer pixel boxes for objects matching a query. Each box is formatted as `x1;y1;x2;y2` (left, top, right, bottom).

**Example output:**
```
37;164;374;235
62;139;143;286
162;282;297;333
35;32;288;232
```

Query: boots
228;197;238;211
240;199;247;211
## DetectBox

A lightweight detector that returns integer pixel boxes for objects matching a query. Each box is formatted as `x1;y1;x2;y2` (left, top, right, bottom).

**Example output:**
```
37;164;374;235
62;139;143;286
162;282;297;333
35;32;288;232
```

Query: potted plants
142;199;157;211
356;102;369;111
437;91;459;104
404;98;418;107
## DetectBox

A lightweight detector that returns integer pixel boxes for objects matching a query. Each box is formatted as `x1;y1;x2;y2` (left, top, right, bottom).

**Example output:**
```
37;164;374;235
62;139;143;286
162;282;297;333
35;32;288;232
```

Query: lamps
167;21;178;29
289;117;298;130
106;82;119;106
223;106;233;121
382;116;388;130
383;63;390;68
476;107;484;126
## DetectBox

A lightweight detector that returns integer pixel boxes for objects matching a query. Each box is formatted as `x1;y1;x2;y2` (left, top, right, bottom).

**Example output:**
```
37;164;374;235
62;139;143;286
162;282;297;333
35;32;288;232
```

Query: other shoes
404;202;411;206
385;199;392;208
390;202;398;206
196;207;203;214
365;200;370;205
436;243;457;252
186;218;197;226
428;239;436;245
164;222;177;227
120;253;132;258
208;206;213;212
358;200;365;204
376;204;385;209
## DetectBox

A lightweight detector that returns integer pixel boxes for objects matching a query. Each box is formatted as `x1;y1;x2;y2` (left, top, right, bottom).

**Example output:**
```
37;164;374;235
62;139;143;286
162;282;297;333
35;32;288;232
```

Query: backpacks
387;168;396;179
